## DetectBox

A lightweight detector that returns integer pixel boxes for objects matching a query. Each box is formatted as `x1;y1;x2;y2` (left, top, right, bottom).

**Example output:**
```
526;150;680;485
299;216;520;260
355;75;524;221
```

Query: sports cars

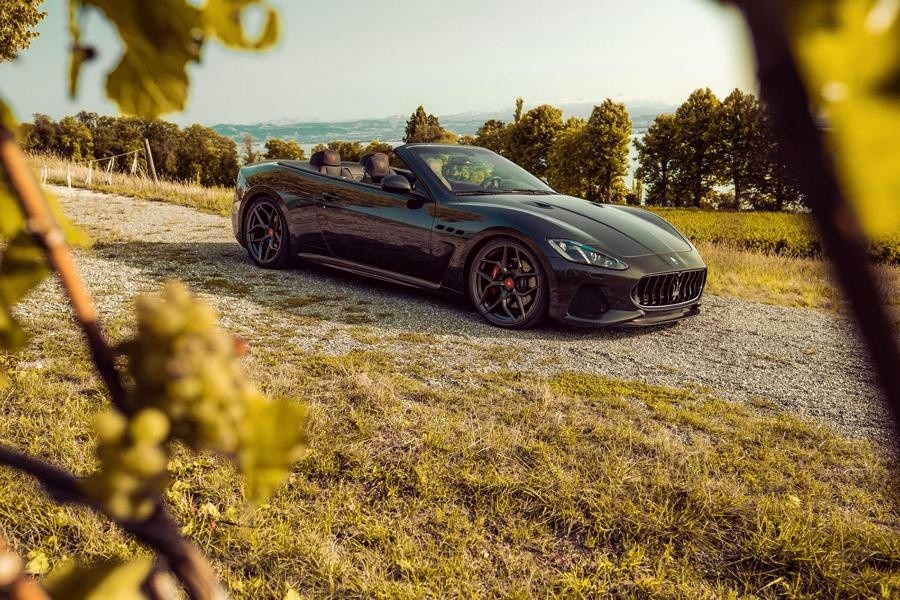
232;145;709;330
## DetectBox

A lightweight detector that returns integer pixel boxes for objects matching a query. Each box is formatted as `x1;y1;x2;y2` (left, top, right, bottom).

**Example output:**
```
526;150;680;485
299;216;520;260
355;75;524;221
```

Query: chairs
360;151;400;185
308;151;354;179
